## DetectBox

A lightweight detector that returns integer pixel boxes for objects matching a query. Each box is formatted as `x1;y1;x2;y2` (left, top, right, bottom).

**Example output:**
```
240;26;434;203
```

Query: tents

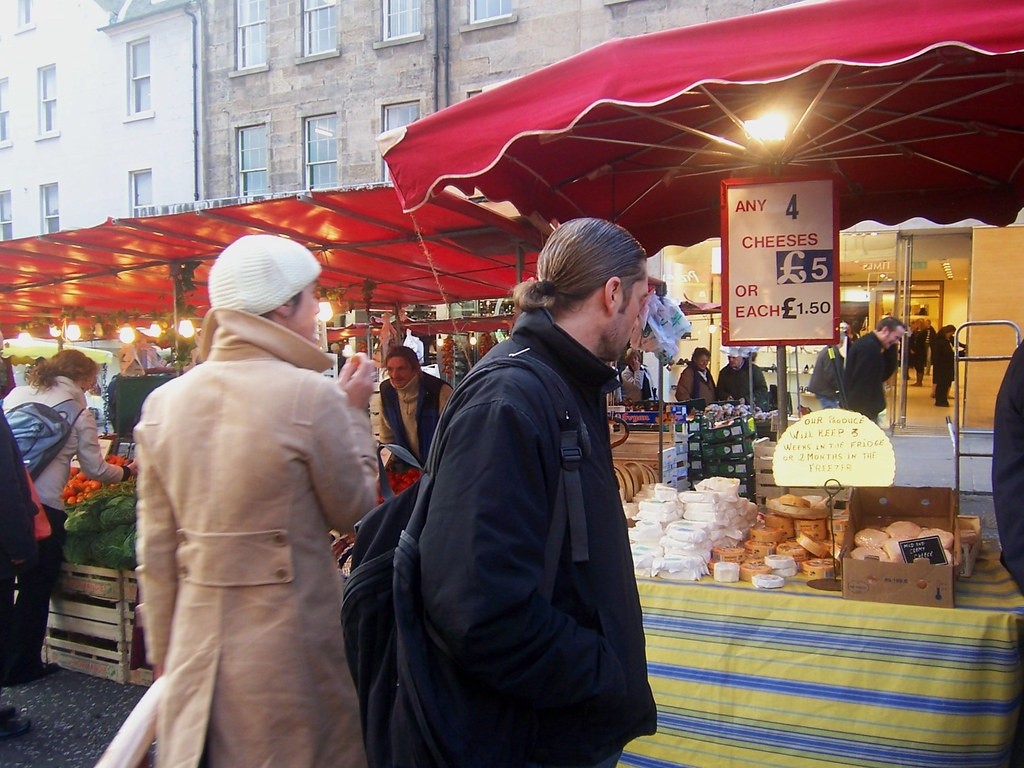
0;332;112;436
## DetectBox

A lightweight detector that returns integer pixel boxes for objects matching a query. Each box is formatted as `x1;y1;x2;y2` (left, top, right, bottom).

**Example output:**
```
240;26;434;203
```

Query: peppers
480;333;492;358
441;337;455;380
87;476;136;503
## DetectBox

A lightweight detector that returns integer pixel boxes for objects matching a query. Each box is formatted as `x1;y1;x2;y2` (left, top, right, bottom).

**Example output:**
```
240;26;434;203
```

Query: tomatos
60;455;126;503
376;468;422;503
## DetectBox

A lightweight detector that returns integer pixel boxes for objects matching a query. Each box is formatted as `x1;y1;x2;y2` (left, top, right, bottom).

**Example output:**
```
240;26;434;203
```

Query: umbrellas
377;1;1024;443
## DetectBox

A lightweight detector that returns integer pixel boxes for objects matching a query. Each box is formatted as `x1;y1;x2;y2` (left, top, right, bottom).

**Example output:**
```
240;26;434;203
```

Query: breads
851;521;953;565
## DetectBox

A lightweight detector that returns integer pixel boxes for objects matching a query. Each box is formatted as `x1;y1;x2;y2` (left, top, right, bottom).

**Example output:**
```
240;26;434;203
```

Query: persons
608;348;655;404
717;353;768;403
1;411;51;738
847;317;906;429
379;347;453;471
1;350;136;688
809;334;844;409
992;340;1024;593
137;233;377;768
910;316;936;387
844;325;858;353
930;324;965;407
395;218;657;768
675;348;721;406
330;342;345;372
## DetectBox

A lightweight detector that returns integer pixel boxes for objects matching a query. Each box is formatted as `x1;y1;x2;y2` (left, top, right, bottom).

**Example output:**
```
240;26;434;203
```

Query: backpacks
336;353;590;768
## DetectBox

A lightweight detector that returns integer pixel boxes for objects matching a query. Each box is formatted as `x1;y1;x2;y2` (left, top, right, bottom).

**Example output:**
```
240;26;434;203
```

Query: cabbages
64;494;137;582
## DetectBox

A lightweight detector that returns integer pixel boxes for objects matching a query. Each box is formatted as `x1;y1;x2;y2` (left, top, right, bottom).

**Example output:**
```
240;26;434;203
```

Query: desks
616;538;1024;768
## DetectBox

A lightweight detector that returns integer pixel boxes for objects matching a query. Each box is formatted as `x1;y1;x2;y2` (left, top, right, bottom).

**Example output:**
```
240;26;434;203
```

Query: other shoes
0;706;31;739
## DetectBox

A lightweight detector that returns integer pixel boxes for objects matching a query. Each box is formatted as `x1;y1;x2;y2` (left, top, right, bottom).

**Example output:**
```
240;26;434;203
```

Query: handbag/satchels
6;398;87;485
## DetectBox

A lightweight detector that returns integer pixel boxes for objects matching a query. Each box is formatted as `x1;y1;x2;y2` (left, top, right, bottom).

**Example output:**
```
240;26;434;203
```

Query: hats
207;236;321;318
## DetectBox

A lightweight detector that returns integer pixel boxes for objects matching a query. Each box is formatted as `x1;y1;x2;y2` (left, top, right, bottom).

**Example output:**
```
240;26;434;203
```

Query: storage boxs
13;560;154;689
117;443;135;461
367;382;381;441
955;514;983;580
610;404;789;514
838;485;960;610
